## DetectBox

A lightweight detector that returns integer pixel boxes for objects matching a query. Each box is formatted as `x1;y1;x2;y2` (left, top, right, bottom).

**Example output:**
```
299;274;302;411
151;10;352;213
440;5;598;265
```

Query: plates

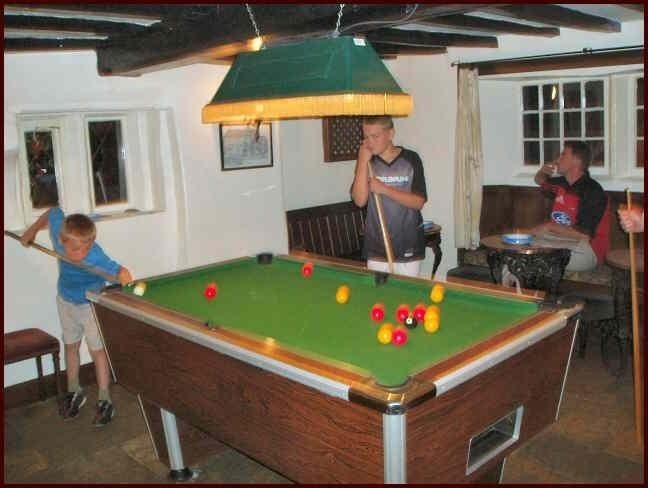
500;233;531;246
422;220;433;229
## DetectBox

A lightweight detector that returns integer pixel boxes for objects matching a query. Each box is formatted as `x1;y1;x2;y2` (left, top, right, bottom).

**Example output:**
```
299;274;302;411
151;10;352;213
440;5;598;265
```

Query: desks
600;250;647;370
422;224;442;278
480;234;570;294
85;250;588;487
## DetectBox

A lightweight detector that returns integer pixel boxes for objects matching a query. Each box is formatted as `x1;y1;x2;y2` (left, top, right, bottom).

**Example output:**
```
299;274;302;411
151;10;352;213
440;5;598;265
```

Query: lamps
203;3;412;124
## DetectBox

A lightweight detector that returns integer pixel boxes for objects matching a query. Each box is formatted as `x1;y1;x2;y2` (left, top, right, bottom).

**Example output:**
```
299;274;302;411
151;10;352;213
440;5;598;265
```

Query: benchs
447;264;624;358
285;200;365;261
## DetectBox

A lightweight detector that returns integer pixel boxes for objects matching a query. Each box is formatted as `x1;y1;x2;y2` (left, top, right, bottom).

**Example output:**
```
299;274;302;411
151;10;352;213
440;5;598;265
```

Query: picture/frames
218;122;274;170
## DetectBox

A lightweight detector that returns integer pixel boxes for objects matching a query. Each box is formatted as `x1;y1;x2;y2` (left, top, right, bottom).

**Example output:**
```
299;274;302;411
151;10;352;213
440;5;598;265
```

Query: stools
3;327;63;407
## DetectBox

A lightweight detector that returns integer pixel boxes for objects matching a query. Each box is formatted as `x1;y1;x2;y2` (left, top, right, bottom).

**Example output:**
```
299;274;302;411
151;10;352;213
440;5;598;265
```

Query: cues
626;188;643;444
362;140;396;272
5;230;132;288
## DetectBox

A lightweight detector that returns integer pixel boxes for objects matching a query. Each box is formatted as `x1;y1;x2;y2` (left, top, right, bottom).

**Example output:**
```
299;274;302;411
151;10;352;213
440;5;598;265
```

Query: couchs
457;186;645;303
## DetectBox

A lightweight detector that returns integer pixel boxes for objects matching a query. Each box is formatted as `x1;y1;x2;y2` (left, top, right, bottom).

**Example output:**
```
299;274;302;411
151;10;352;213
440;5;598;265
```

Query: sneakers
63;391;87;422
92;400;116;427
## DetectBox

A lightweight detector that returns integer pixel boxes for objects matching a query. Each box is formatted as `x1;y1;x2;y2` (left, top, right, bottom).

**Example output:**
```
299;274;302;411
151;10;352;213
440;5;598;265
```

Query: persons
617;208;644;233
350;115;427;277
502;140;612;287
20;206;132;427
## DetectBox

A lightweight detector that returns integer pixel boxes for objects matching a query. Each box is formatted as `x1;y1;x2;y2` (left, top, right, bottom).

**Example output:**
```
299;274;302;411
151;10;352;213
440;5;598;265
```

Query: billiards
303;264;314;275
134;284;146;296
205;287;217;298
335;289;350;303
369;283;446;348
